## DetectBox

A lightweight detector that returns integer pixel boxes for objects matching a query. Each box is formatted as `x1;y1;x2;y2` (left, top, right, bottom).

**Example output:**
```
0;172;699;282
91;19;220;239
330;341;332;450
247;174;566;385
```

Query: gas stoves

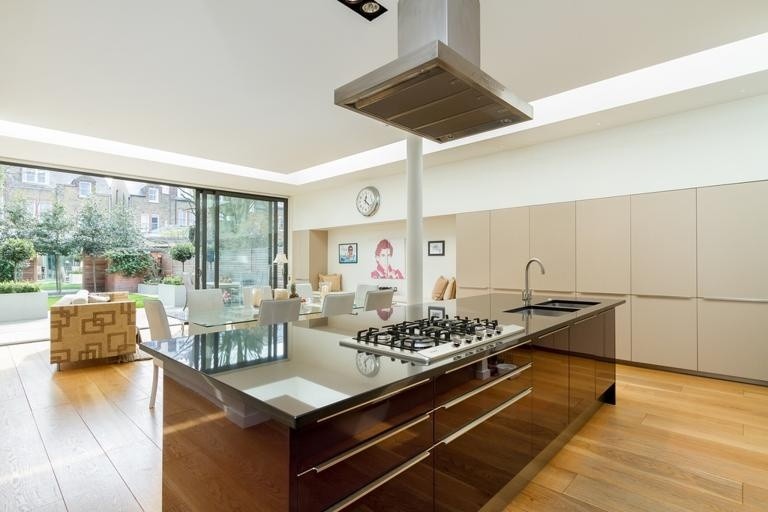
338;315;526;363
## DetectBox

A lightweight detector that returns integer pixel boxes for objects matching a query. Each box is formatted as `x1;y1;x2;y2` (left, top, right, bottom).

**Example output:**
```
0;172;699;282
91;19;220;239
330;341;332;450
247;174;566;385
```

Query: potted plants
0;281;49;324
159;276;186;308
137;278;159;295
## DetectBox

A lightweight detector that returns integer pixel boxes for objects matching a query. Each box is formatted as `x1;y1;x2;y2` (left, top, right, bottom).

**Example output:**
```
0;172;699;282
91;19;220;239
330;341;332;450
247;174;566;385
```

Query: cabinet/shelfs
456;205;530;299
290;337;532;512
631;179;767;384
596;308;619;400
530;195;631;361
532;312;596;459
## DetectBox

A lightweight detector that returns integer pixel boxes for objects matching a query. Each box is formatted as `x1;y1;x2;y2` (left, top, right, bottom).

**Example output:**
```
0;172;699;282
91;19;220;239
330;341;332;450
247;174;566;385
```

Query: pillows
443;276;456;300
72;289;111;303
431;275;448;301
318;272;342;292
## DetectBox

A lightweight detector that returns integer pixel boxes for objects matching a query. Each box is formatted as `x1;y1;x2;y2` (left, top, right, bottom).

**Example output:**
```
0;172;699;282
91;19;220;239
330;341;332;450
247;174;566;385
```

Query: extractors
333;0;534;144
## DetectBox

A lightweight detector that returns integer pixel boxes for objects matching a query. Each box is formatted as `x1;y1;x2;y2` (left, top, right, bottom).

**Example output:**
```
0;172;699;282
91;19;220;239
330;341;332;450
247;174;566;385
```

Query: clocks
355;186;380;217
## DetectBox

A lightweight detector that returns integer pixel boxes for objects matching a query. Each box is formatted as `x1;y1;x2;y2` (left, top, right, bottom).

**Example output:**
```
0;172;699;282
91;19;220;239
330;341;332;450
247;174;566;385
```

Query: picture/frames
338;242;358;264
427;239;446;256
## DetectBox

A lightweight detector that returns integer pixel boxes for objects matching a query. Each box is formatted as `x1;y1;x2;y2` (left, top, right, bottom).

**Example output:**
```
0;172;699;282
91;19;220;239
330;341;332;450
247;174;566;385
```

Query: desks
165;301;364;328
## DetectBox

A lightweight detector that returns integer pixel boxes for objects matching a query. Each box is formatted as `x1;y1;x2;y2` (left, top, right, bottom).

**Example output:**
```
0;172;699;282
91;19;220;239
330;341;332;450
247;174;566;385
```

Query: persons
347;246;356;262
377;308;394;321
371;240;403;279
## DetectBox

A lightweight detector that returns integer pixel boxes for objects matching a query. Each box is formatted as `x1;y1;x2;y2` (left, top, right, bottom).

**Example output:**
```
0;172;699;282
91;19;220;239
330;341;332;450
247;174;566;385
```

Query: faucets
523;257;545;305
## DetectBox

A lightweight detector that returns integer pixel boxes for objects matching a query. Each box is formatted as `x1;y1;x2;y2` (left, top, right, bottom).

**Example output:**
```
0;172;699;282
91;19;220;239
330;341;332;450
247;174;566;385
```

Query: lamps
272;253;290;275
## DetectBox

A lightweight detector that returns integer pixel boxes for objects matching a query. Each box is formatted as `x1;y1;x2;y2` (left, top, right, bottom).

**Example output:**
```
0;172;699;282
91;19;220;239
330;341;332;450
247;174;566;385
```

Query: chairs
322;291;355;314
143;299;173;410
188;288;227;336
242;285;272;305
258;298;301;322
364;289;394;309
49;291;137;372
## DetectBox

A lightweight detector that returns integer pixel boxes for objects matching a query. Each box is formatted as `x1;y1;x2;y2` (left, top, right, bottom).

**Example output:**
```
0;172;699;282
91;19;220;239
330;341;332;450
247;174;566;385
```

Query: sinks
533;298;601;310
505;305;581;318
